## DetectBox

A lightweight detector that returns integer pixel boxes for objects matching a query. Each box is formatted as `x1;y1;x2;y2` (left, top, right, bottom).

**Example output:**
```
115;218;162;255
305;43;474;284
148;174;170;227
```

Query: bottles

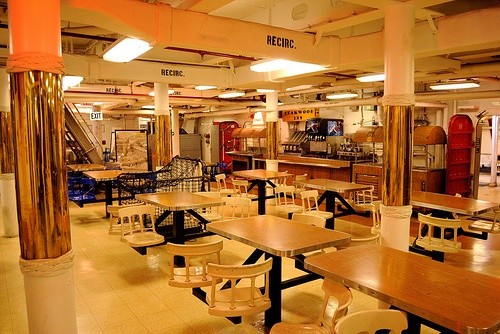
372;116;375;125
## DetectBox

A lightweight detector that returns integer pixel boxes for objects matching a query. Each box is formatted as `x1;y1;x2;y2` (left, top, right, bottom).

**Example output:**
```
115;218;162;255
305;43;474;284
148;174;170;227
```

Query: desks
203;163;220;182
293;179;372;231
68;163;104;198
83;170;127;220
406;186;499;259
302;243;499;334
207;215;352;334
232;169;295;216
132;192;226;247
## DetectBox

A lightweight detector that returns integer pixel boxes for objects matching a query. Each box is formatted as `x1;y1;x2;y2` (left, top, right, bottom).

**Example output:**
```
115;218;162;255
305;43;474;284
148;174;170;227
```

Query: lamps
326;93;360;101
219;91;245;100
356;74;387;84
148;88;175;98
428;80;483;91
103;36;152;63
196;85;217;93
249;57;302;73
62;75;84;93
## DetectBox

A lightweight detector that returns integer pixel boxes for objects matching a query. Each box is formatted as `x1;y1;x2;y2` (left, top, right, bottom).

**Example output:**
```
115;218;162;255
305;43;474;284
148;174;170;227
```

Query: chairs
107;174;500;334
